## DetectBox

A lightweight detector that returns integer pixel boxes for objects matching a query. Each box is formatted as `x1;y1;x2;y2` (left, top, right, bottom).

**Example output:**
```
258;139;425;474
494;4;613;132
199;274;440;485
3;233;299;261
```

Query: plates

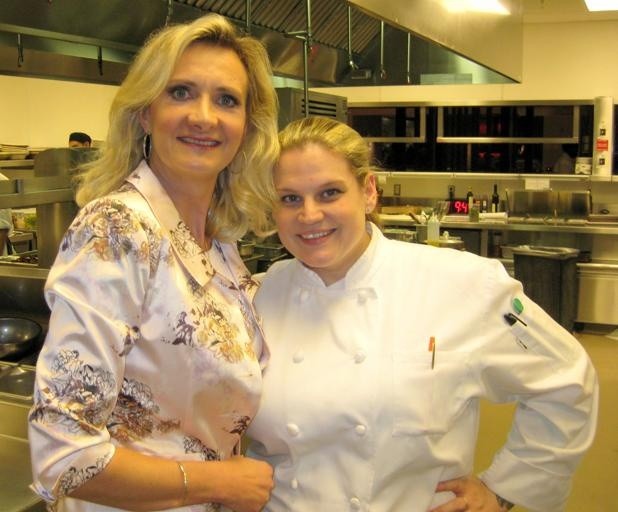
0;144;45;160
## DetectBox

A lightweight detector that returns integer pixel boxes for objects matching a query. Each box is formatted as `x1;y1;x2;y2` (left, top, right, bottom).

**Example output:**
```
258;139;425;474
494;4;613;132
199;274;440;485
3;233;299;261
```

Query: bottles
428;213;440;247
467;184;500;214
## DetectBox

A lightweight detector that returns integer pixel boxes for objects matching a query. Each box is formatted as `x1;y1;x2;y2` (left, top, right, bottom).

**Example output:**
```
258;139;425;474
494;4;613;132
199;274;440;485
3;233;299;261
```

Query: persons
69;131;92;148
27;11;274;512
241;116;601;512
0;173;17;258
553;144;578;173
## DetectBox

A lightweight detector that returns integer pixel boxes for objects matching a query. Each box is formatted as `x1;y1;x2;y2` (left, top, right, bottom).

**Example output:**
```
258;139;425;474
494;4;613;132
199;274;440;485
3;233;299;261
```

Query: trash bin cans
512;244;580;335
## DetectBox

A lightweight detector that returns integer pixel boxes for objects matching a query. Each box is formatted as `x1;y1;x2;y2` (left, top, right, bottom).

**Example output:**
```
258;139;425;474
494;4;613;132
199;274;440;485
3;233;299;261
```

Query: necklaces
196;239;208;253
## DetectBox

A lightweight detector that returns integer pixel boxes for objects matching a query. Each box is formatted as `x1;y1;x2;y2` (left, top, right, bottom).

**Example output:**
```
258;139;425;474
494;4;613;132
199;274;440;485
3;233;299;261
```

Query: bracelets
176;462;189;507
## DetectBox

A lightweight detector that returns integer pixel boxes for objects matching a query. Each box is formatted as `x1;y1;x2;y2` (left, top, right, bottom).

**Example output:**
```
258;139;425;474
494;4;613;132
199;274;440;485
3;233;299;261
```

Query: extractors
172;0;382;86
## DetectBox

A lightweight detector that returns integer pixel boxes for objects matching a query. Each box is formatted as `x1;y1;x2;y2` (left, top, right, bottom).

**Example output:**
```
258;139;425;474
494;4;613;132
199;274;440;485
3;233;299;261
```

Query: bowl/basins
414;224;429;244
0;316;43;361
